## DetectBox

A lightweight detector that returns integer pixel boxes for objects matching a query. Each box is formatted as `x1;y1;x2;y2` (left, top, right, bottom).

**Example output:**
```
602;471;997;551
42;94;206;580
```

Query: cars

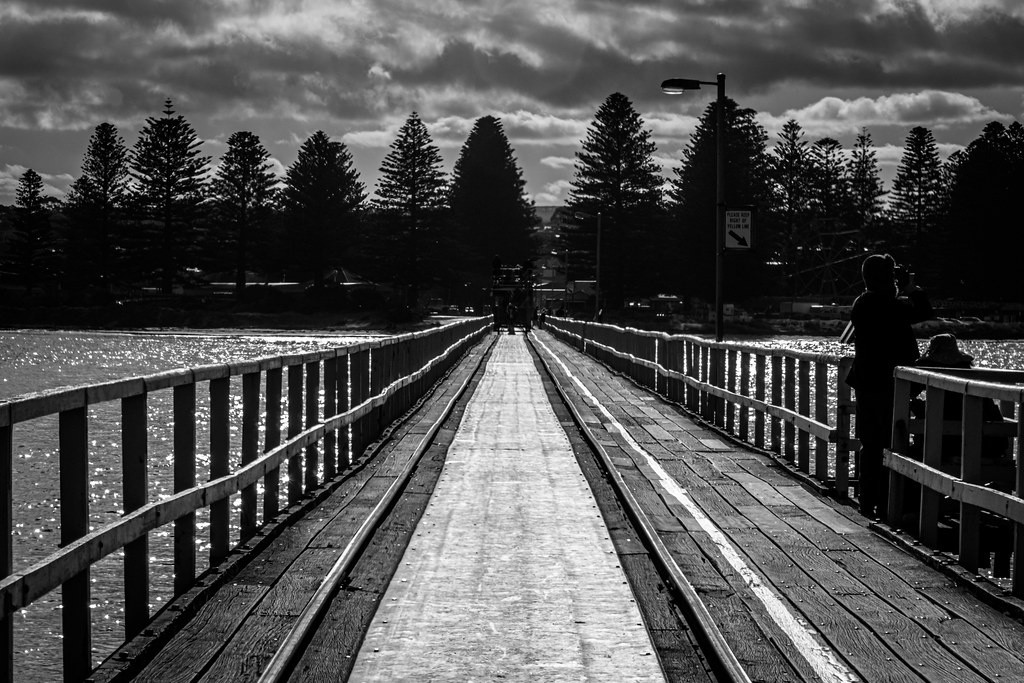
808;303;986;334
448;303;493;317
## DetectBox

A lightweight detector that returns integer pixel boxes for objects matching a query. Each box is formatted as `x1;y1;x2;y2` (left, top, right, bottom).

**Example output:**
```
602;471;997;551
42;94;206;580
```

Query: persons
848;253;938;514
914;332;1012;530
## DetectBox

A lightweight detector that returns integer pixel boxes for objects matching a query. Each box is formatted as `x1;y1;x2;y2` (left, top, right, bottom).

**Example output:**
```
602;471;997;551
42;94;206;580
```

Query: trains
487;264;534;334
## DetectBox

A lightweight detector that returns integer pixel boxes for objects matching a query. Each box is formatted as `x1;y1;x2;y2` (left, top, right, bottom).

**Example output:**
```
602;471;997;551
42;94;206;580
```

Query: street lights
660;73;725;343
575;211;602;322
531;233;569;319
